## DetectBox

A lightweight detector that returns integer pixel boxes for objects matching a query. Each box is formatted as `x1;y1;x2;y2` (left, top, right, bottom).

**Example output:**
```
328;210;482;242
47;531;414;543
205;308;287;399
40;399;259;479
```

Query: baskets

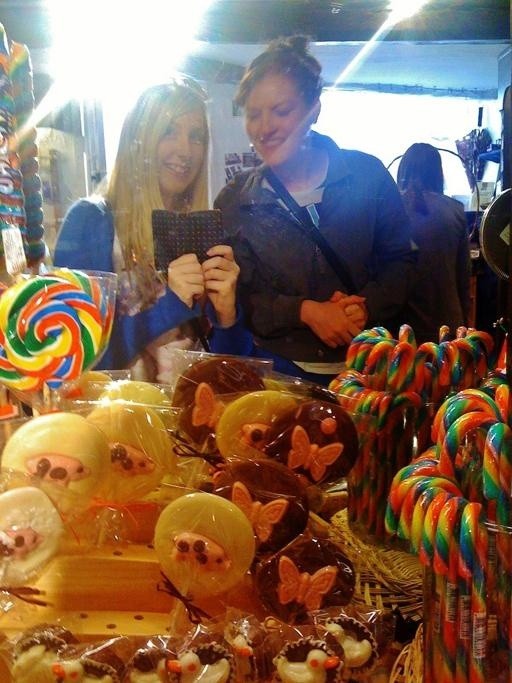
327;508;427;623
388;616;501;683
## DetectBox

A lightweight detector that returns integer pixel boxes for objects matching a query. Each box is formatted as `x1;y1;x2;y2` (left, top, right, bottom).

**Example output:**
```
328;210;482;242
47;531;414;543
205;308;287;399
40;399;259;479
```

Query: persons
396;142;474;344
211;34;416;374
51;81;256;369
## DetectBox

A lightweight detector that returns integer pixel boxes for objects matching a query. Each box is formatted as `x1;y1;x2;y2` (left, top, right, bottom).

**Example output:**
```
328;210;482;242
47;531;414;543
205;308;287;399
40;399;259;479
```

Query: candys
0;43;512;683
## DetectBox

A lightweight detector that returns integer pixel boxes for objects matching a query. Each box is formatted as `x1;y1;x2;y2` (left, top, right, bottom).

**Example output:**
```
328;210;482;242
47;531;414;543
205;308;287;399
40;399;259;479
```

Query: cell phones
188;209;224;265
